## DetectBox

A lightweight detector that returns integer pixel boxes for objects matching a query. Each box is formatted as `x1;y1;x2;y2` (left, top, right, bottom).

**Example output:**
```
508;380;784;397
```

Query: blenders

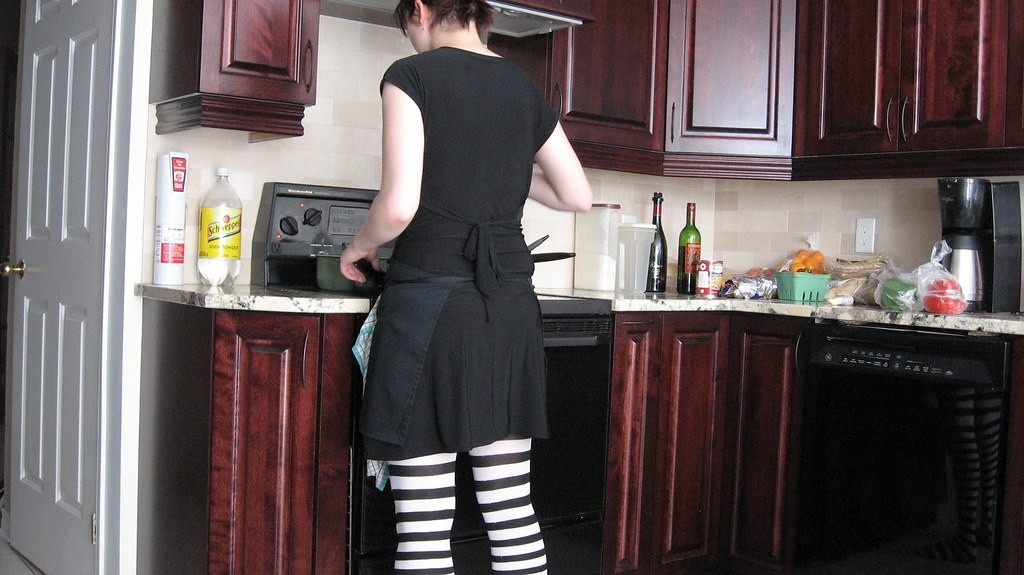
934;177;1022;313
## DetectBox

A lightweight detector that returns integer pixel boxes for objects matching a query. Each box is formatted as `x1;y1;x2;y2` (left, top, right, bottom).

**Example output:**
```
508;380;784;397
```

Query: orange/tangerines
776;248;830;273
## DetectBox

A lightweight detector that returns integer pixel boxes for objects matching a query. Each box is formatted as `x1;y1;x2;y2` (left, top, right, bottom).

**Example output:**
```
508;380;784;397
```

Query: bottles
676;202;701;296
196;167;242;287
645;191;668;292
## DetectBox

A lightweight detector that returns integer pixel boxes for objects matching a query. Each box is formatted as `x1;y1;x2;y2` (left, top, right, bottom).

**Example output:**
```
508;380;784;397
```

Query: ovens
355;317;614;575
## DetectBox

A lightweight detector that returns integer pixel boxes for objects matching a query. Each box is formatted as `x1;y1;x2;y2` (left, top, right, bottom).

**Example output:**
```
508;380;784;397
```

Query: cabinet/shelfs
670;0;794;159
792;0;1008;157
208;312;351;574
604;312;729;575
147;0;320;106
715;310;810;574
487;0;670;175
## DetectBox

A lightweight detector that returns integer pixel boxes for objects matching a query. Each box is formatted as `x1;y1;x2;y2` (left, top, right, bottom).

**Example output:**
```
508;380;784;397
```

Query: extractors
318;0;585;40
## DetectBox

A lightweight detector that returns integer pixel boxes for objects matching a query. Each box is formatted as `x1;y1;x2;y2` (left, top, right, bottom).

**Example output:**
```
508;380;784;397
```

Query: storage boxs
775;270;831;303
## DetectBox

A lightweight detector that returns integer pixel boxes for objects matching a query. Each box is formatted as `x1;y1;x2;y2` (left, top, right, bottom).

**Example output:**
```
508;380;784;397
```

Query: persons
341;0;591;575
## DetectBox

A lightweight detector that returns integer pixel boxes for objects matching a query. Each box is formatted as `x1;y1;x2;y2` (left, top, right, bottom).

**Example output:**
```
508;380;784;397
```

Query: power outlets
854;217;875;254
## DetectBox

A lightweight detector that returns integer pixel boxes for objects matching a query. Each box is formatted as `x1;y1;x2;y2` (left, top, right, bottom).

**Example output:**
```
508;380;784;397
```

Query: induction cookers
252;179;613;317
